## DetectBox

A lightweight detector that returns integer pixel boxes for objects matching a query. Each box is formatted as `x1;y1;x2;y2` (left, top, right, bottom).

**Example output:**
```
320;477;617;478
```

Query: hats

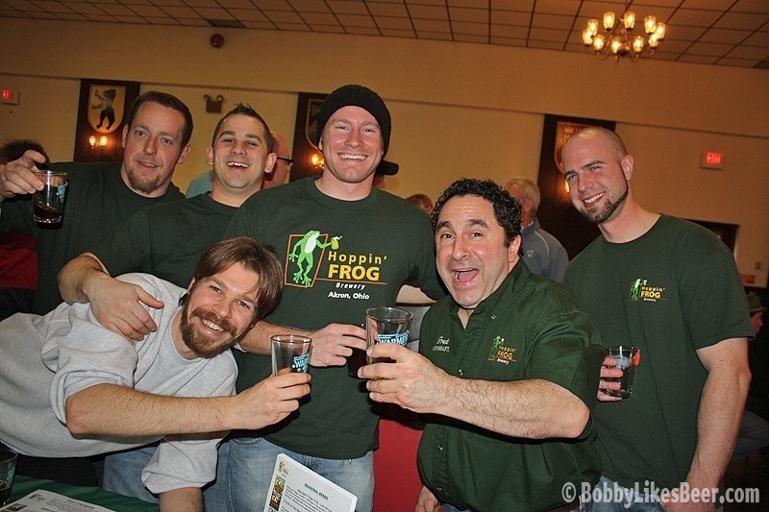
374;159;398;176
747;291;763;313
316;85;392;155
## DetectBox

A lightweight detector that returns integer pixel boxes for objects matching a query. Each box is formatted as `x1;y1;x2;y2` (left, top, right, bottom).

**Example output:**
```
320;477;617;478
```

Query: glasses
277;156;295;167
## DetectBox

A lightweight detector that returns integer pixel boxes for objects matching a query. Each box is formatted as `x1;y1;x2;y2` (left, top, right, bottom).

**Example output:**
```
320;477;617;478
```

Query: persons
560;126;756;512
56;102;278;512
0;139;51;320
372;159;399;191
504;177;570;284
223;83;450;512
356;176;606;512
1;90;194;312
405;193;433;214
262;130;295;190
0;237;312;512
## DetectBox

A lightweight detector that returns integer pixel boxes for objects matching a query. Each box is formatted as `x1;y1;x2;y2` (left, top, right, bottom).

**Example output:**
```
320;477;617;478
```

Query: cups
608;344;636;398
366;306;413;365
270;335;312;376
347;325;366;378
31;170;67;224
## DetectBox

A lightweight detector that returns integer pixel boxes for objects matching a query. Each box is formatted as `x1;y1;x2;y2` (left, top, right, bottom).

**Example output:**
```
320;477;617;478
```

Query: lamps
89;136;108;153
581;0;668;65
